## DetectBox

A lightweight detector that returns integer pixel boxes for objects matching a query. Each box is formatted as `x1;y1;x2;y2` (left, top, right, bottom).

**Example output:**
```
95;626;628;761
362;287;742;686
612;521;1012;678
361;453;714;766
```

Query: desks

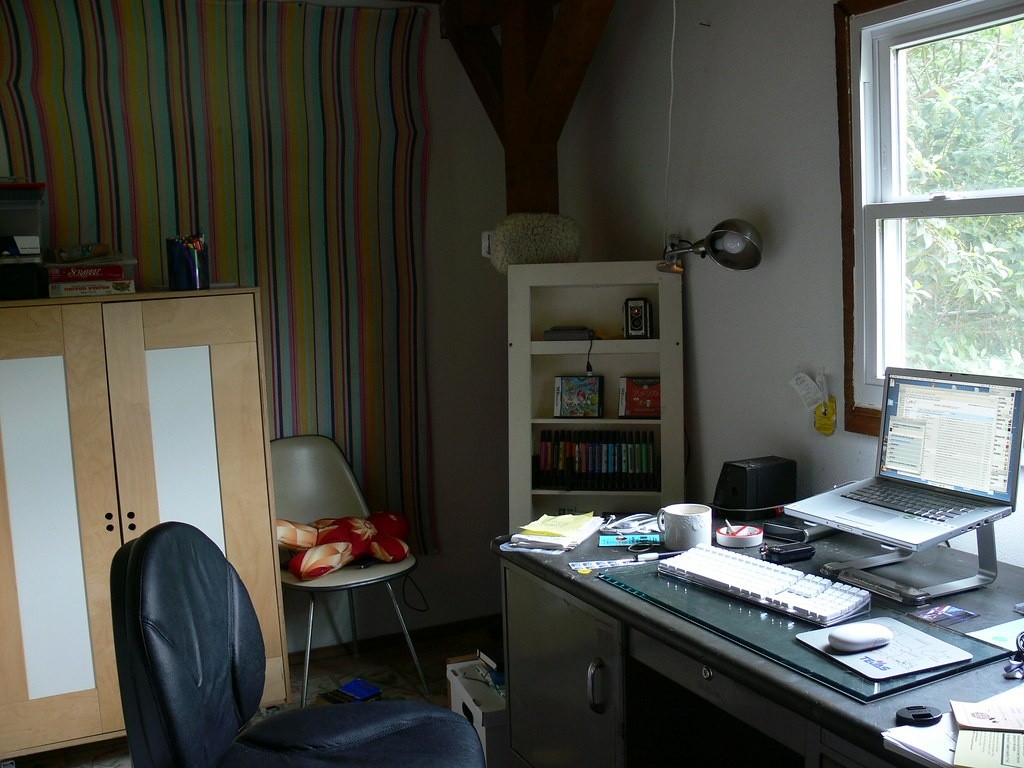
490;513;1024;768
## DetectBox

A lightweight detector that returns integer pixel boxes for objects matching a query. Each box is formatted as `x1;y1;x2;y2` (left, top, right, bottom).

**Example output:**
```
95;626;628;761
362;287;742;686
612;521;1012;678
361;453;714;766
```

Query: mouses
828;623;892;652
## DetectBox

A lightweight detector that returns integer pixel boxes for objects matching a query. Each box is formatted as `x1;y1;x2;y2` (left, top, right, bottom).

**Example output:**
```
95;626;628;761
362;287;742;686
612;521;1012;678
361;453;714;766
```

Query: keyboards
658;543;872;628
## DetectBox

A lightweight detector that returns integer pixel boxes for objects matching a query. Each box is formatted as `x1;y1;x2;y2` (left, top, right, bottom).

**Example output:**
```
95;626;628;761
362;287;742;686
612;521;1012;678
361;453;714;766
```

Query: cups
165;237;209;290
657;504;711;551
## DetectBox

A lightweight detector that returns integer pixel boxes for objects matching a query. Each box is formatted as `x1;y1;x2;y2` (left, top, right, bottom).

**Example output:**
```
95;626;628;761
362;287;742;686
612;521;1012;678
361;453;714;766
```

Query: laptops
784;366;1024;552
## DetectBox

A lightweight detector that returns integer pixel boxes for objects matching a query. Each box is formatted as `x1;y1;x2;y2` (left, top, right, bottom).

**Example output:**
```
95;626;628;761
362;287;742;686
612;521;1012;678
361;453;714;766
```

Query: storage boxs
445;649;525;768
0;250;53;301
0;190;50;257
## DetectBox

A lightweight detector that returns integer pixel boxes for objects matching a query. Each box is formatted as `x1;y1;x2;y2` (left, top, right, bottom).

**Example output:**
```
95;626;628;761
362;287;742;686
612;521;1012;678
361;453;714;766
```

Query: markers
167;232;210;289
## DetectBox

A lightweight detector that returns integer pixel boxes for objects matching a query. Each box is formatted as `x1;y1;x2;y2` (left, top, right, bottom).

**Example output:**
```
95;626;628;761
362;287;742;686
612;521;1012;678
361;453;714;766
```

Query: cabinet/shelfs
0;289;292;761
507;259;684;537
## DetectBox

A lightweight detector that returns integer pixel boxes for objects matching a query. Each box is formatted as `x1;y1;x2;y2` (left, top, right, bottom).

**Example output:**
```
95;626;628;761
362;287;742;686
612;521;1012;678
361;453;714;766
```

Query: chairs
271;435;431;709
127;521;488;768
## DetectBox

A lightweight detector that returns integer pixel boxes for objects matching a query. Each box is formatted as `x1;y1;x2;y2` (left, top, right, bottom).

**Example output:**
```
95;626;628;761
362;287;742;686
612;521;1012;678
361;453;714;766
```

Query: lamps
655;219;763;274
